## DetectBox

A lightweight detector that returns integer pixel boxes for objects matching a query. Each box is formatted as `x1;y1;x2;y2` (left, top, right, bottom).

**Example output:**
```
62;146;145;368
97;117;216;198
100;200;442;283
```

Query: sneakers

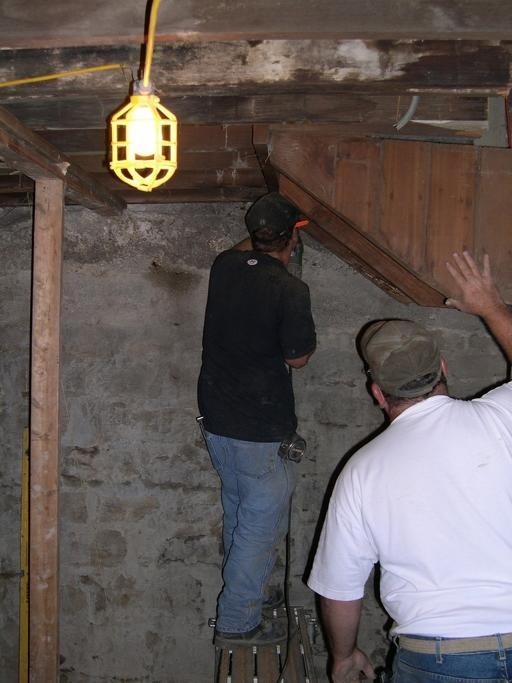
214;584;287;649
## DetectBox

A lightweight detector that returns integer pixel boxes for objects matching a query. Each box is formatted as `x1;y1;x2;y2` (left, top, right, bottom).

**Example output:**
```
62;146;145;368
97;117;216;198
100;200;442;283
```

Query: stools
218;606;317;683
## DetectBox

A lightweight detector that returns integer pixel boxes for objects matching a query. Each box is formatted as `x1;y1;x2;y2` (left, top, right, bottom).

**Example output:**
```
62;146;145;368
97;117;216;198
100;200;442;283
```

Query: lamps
108;0;178;191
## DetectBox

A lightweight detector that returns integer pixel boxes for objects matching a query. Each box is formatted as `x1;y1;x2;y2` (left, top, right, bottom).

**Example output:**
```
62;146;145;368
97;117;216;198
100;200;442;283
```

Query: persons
197;192;316;646
306;251;512;683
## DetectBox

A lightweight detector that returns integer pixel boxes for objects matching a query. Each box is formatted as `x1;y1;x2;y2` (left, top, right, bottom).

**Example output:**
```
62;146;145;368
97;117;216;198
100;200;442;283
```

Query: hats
245;192;309;231
360;321;442;398
